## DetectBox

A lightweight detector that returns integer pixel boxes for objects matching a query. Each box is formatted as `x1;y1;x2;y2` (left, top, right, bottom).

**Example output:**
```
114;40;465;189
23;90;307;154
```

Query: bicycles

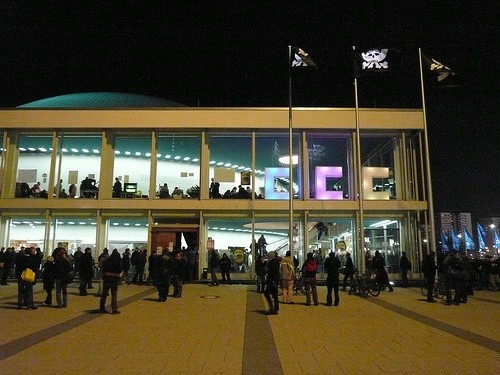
253;273;268;285
348;273;380;297
293;277;314;294
421;273;446;299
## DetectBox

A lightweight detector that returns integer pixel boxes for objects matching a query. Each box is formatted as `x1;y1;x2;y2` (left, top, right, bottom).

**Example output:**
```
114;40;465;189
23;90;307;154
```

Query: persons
29;177;263;199
0;234;500;315
290;223;299;246
314;222;330;239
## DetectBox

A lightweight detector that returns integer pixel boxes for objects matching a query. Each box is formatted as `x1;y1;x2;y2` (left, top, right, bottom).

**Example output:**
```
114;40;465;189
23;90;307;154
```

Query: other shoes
27;306;38;310
216;279;220;285
208;283;215;285
112;310;121;314
170;294;176;296
175;294;181;298
43;303;50;306
53;305;66;308
99;310;109;313
264;309;278;315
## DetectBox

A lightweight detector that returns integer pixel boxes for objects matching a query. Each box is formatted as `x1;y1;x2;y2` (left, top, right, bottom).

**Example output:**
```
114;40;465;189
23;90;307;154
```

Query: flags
354;49;399;75
292;48;316;68
422;54;457;86
440;222;500;252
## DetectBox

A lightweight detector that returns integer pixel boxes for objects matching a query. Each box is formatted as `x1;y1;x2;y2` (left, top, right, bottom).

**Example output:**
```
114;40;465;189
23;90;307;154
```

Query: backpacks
303;259;316;271
64;267;76;284
448;254;460;274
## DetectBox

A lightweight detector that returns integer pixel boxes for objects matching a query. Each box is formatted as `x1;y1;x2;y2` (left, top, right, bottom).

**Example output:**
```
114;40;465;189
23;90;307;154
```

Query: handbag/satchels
21;268;36;283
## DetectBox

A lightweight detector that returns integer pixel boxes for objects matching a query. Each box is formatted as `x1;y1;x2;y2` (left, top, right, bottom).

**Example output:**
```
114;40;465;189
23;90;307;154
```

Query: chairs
16;182;257;199
